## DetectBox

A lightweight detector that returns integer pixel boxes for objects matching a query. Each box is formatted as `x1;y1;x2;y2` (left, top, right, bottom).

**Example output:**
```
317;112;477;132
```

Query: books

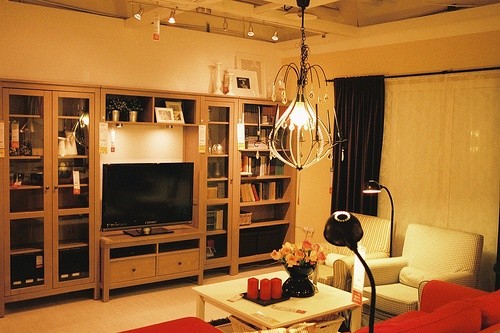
207;182;226;199
245;126;287;149
208;158;229;178
241;154;285;177
206;208;224;231
240;181;283;202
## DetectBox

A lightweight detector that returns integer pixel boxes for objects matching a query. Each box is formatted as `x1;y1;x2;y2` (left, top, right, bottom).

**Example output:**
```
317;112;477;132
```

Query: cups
271;278;282;299
248;278;258;300
260;279;271;299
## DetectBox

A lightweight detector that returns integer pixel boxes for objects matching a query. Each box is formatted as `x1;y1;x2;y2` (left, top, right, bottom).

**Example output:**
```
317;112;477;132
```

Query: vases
282;263;317;299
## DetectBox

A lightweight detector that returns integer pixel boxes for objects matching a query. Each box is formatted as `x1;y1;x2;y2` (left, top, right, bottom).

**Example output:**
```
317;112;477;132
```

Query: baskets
229;315;345;333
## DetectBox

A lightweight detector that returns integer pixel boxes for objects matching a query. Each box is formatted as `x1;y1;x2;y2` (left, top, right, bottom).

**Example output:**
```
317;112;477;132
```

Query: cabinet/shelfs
101;224;202;303
0;77;101;320
230;95;297;275
196;93;237;275
101;85;202;126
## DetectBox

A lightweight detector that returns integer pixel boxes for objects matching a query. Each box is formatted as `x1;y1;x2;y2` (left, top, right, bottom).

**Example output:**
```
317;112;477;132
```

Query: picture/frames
227;69;260;98
165;102;186;124
154;106;175;123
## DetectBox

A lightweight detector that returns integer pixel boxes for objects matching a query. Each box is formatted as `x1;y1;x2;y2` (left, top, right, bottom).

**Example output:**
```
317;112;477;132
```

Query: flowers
271;239;326;267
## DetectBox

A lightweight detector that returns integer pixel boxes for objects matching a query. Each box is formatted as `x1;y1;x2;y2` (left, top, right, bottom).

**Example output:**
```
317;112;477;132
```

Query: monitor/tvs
101;162;193;237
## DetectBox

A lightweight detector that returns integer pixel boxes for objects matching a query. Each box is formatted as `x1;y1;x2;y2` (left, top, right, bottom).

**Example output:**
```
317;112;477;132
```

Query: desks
193;270;369;333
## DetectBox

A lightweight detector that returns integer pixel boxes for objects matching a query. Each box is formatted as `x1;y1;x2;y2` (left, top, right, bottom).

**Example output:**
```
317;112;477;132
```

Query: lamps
323;211;376;333
267;0;332;171
271;31;279;41
248;22;254;37
134;7;145;21
362;180;394;256
168;9;177;24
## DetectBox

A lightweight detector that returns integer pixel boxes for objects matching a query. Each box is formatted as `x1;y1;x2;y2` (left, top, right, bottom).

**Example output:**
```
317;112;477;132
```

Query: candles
269;278;283;300
247;277;259;300
260;279;271;300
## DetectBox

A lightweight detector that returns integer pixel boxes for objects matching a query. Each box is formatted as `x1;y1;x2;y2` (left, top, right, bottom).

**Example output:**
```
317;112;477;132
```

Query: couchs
361;223;483;326
313;211;390;292
115;315;225;333
354;279;500;333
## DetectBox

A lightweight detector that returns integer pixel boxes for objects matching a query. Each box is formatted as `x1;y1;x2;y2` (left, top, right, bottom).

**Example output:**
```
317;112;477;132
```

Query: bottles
58;136;66;155
66;132;78;155
